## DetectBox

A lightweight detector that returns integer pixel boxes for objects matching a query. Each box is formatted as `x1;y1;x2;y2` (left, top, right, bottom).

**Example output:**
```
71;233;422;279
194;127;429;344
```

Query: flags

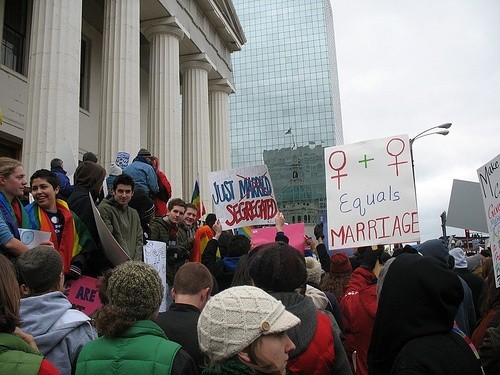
191;182;206;220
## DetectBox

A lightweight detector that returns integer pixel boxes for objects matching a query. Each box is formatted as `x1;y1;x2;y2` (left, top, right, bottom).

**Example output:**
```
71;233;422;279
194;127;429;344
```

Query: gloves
314;223;325;240
68;262;83;280
357;245;384;271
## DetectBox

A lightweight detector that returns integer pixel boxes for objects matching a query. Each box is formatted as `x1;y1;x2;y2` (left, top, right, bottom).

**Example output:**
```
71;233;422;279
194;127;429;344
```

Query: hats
247;240;308;291
100;260;164;316
228;235;252;255
197;284;302;360
330;252;352;273
109;163;123;176
303;256;325;285
138;149;153;158
19;246;62;289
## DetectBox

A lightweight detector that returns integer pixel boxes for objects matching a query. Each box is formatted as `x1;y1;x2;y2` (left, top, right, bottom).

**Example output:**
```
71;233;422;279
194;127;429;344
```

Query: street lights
407;122;452;244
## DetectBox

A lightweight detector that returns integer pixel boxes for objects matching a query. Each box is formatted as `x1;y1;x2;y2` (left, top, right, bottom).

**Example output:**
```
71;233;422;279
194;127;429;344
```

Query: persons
98;174;144;263
83;152;104;207
14;247;98;375
413;238;500;375
303;222;417;375
106;148;172;232
25;168;97;286
153;261;211;375
365;252;487;375
50;158;74;202
148;198;289;292
71;261;198;375
247;240;354;375
68;161;114;279
0;255;61;375
197;284;302;375
0;157;55;264
